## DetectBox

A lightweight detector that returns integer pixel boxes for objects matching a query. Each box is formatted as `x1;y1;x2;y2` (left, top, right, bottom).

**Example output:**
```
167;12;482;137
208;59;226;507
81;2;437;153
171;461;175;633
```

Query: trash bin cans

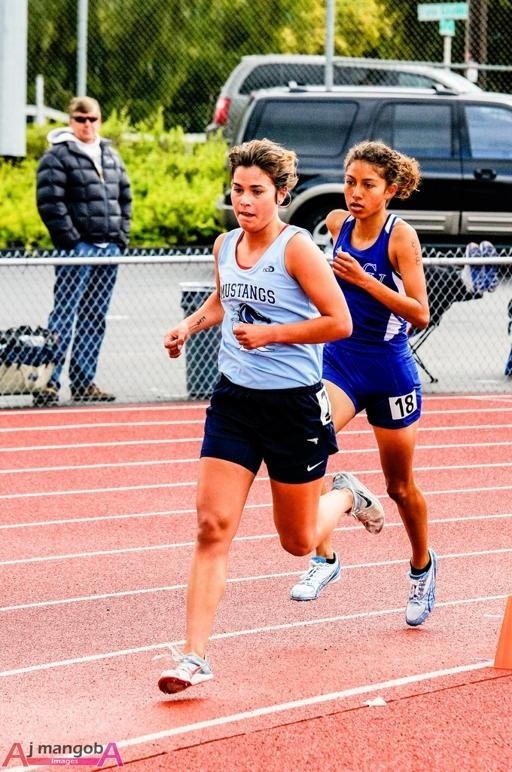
180;281;222;401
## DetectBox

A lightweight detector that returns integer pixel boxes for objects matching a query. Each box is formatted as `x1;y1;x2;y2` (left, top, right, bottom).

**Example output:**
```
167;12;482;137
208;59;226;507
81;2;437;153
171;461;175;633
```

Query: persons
148;125;387;693
286;141;438;630
404;238;499;337
30;94;136;408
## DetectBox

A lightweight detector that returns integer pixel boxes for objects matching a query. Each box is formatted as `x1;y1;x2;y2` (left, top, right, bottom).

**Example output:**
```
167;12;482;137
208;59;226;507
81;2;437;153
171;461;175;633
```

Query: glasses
70;116;99;122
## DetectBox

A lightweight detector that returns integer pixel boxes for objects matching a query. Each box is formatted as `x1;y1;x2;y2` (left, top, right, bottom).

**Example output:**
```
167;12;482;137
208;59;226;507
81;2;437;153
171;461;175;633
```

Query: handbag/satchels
0;325;61;395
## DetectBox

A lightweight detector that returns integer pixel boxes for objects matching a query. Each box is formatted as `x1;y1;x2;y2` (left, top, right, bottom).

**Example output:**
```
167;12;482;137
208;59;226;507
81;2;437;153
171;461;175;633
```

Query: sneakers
290;551;341;601
331;473;384;535
150;647;214;694
405;548;436;626
461;241;496;293
32;388;58;402
74;385;115;401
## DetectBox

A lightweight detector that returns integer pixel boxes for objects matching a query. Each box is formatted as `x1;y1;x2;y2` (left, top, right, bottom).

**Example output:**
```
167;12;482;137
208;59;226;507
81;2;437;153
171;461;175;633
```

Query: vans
208;55;484;146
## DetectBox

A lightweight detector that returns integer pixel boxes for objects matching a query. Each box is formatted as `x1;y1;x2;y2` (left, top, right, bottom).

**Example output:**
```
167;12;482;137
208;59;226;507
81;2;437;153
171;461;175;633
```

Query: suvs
224;80;511;244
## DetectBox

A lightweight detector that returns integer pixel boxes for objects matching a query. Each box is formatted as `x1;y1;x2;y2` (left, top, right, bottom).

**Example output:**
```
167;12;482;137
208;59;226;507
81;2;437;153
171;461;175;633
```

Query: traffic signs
418;5;468;25
